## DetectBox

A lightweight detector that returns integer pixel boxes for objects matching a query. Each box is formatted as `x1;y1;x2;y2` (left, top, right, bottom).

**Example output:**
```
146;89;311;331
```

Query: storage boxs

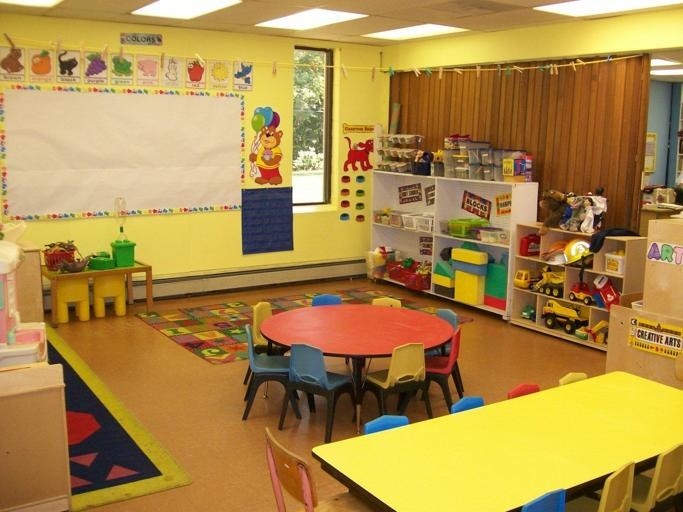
441;135;534;182
603;251;623;275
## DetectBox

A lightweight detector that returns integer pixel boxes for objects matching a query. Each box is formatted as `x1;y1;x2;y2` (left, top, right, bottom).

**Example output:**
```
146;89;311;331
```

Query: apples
188;61;204;81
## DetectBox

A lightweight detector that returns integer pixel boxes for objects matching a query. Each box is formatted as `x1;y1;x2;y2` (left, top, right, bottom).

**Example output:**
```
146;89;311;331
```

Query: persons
579;198;595;233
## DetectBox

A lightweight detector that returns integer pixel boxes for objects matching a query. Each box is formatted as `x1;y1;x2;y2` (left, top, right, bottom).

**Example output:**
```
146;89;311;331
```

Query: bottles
112;197;130;242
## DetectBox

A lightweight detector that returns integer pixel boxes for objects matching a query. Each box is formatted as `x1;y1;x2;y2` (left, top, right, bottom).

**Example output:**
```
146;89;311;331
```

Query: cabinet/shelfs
673;87;682;186
509;221;647;354
367;168;540;322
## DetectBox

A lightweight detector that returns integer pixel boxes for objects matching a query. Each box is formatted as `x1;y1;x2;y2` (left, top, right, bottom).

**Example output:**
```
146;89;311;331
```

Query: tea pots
60;255;94;272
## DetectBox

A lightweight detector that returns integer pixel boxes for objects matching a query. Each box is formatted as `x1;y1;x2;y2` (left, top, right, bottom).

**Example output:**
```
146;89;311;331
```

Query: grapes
84;58;107;76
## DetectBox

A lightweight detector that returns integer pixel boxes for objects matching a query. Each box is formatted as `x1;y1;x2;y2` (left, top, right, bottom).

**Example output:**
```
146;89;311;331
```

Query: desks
40;260;153;314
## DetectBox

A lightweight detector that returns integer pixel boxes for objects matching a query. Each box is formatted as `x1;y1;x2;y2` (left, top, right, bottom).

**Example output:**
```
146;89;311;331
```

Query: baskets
43;251;77;272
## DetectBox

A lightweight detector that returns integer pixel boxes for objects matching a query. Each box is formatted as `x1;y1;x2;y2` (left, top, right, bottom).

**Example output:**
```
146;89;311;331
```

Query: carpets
133;282;474;366
43;321;194;511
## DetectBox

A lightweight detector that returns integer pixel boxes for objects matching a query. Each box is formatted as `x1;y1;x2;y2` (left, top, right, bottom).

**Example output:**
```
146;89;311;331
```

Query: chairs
54;277;90;324
241;293;464;442
266;370;683;512
92;273;127;318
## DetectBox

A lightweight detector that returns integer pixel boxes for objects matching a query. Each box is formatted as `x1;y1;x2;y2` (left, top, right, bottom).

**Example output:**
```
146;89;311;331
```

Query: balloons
249;106;282;133
